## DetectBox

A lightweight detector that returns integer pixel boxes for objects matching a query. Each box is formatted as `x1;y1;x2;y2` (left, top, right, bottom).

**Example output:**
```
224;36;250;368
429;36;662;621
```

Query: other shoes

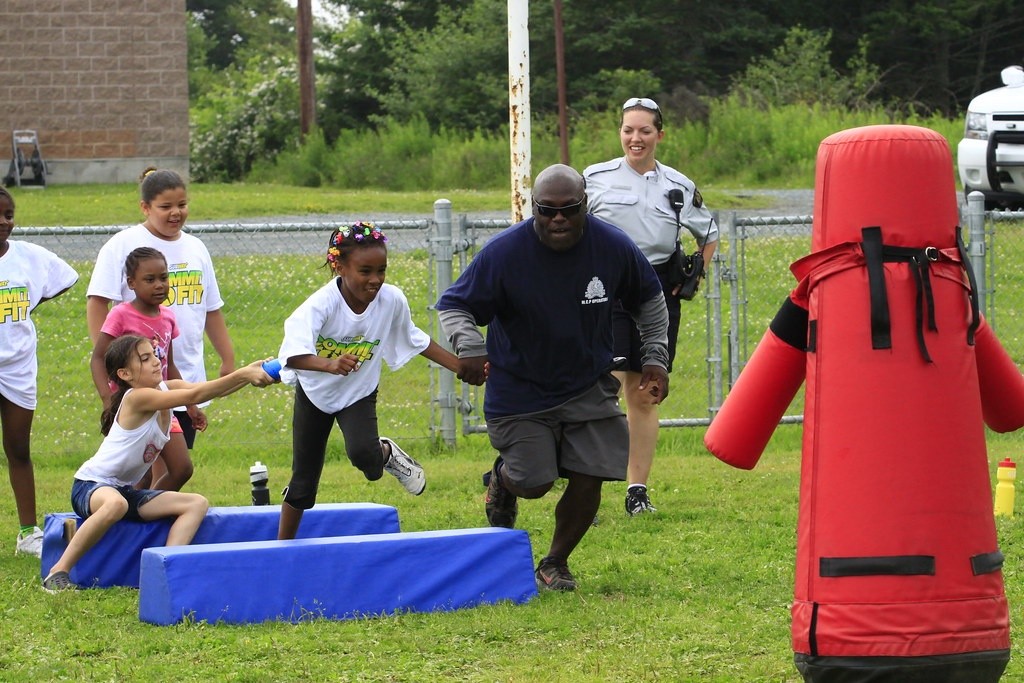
42;570;80;597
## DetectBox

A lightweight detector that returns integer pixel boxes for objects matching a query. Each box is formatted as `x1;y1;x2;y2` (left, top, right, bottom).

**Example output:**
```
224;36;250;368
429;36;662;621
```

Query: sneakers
15;526;44;558
484;454;518;532
624;485;658;519
535;554;579;593
379;437;427;496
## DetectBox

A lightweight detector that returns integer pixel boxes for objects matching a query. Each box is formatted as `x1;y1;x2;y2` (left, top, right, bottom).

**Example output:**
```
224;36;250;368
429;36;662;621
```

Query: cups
251;359;282;387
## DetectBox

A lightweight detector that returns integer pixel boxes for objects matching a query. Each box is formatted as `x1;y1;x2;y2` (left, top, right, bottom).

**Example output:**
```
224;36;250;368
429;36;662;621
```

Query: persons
86;164;234;495
0;185;81;562
433;162;671;594
277;219;490;540
581;97;719;517
38;333;284;596
89;246;209;493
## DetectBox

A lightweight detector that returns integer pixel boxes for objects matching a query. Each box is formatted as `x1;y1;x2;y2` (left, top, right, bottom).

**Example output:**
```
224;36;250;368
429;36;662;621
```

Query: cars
955;65;1024;215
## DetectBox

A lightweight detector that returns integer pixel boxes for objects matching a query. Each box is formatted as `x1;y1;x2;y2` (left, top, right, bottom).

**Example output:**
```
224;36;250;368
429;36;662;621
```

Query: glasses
531;195;586;217
622;97;662;123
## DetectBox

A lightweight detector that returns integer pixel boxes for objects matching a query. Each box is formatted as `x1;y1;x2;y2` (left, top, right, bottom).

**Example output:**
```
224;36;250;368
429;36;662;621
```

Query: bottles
250;461;270;506
